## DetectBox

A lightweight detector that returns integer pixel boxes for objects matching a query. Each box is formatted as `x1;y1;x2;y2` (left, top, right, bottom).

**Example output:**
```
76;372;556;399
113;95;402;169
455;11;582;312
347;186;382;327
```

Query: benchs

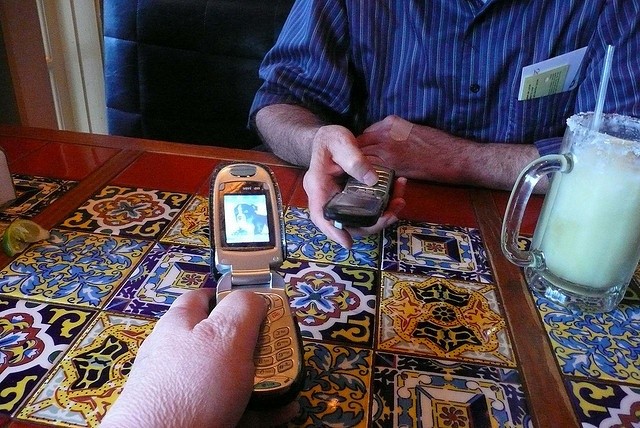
101;0;293;156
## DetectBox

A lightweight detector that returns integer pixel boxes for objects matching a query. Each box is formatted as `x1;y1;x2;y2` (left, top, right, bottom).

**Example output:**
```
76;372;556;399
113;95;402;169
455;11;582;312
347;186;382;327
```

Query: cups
500;109;640;317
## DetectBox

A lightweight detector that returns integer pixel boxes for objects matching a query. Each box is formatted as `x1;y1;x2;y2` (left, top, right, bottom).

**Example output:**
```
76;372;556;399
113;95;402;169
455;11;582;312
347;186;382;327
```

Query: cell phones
322;163;395;225
205;161;303;397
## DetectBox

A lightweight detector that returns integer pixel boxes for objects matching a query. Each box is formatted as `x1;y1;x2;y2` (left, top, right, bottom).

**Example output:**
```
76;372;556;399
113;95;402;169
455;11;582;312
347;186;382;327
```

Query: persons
94;284;268;428
249;1;640;251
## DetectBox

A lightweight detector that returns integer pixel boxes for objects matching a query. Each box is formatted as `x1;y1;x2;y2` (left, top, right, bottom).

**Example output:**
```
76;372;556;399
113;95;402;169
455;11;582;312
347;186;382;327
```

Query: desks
0;121;640;426
